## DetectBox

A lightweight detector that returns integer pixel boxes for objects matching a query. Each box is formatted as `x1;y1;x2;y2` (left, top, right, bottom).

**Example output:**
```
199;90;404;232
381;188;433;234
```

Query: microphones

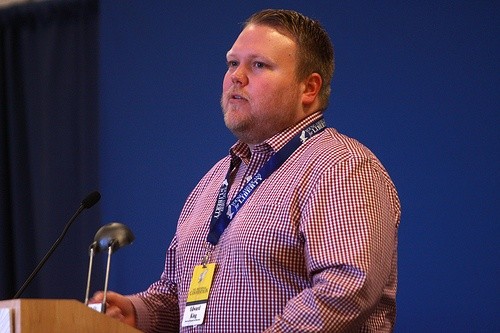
14;191;102;299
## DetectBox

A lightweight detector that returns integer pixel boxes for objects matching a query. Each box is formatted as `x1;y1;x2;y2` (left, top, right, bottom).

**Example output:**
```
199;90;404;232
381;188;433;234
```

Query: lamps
85;222;135;313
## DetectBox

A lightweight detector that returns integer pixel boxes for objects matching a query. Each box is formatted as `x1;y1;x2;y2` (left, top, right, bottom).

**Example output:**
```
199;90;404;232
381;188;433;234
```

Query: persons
87;8;401;333
